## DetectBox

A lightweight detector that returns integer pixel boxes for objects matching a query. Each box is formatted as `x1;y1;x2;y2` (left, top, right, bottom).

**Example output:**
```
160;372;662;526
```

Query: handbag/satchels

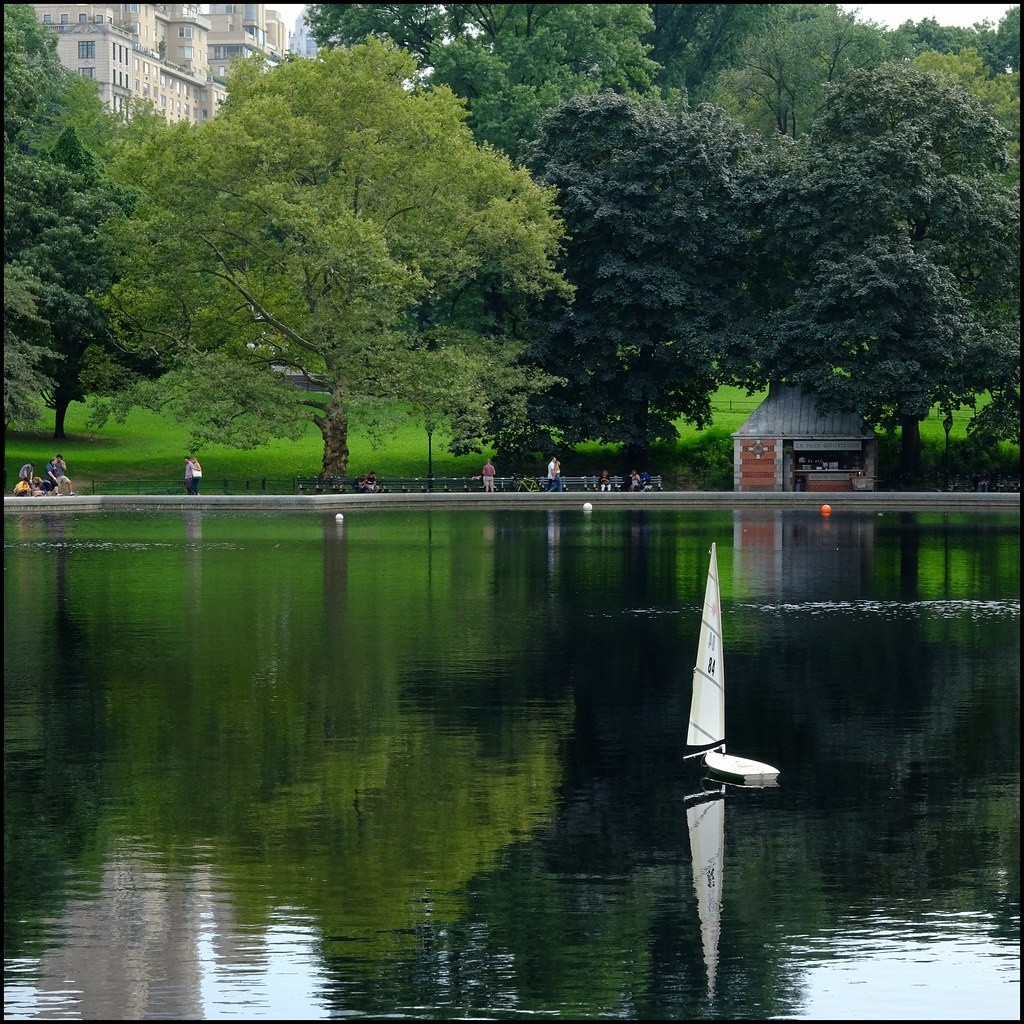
191;463;202;478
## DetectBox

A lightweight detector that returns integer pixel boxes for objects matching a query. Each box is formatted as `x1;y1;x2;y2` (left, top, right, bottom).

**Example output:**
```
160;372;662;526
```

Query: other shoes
69;492;75;496
55;493;62;496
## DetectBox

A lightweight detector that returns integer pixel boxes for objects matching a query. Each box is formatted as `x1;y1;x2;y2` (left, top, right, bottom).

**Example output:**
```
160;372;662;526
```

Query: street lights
943;416;953;492
425;422;435;491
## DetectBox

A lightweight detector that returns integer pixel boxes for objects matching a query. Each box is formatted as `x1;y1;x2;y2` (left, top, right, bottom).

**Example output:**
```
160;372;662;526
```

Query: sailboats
683;783;726;1005
689;541;780;788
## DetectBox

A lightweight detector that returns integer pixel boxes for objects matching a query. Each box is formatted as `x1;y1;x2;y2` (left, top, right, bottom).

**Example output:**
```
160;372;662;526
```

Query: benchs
297;475;664;495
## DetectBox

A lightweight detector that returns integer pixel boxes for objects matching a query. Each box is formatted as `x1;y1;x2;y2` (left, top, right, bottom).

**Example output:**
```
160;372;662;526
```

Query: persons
547;457;563;492
353;474;369;492
190;457;201;495
46;458;62;496
54;454;75;496
184;456;196;495
630;470;640;492
598;470;612;491
13;477;47;497
364;471;380;493
19;462;36;489
483;459;496;491
